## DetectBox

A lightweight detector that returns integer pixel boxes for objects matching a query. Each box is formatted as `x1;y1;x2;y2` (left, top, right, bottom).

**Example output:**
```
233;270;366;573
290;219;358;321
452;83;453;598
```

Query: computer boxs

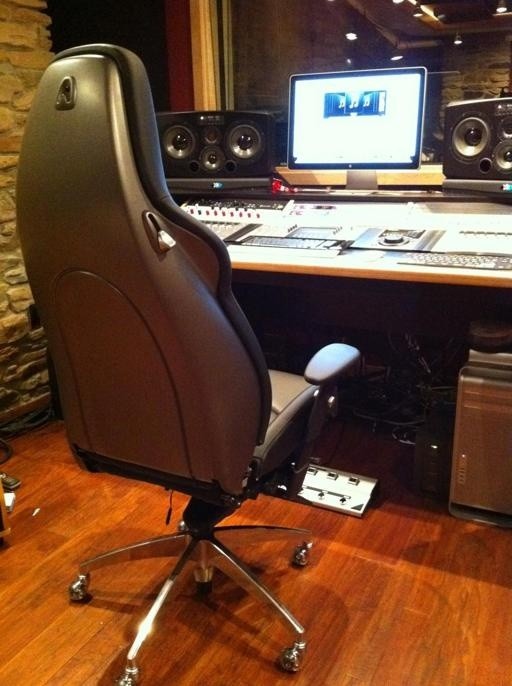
447;347;512;531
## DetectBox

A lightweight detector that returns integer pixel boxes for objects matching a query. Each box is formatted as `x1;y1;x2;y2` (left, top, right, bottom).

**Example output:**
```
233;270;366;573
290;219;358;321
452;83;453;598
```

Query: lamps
495;0;508;14
453;23;463;46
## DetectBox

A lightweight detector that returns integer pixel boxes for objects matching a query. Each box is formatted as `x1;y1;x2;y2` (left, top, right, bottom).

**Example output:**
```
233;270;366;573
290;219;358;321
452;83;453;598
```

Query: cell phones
0;472;23;491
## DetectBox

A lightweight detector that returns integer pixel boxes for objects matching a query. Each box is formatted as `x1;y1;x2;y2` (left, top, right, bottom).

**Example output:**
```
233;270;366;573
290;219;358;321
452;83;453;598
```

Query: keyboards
396;252;512;271
242;237;344;251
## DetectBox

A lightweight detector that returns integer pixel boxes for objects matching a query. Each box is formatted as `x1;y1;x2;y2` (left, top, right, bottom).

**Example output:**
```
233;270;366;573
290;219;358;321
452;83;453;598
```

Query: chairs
13;41;361;686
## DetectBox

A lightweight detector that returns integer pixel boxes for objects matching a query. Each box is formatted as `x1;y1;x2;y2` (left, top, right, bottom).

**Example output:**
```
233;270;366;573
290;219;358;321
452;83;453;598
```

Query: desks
174;197;512;288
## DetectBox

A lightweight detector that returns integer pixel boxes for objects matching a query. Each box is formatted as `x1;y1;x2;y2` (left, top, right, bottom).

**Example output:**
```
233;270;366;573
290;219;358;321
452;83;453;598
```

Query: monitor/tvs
286;64;427;190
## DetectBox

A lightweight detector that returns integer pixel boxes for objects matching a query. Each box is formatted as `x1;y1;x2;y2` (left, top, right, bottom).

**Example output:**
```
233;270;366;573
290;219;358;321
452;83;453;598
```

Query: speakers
442;98;512;180
155;110;280;180
